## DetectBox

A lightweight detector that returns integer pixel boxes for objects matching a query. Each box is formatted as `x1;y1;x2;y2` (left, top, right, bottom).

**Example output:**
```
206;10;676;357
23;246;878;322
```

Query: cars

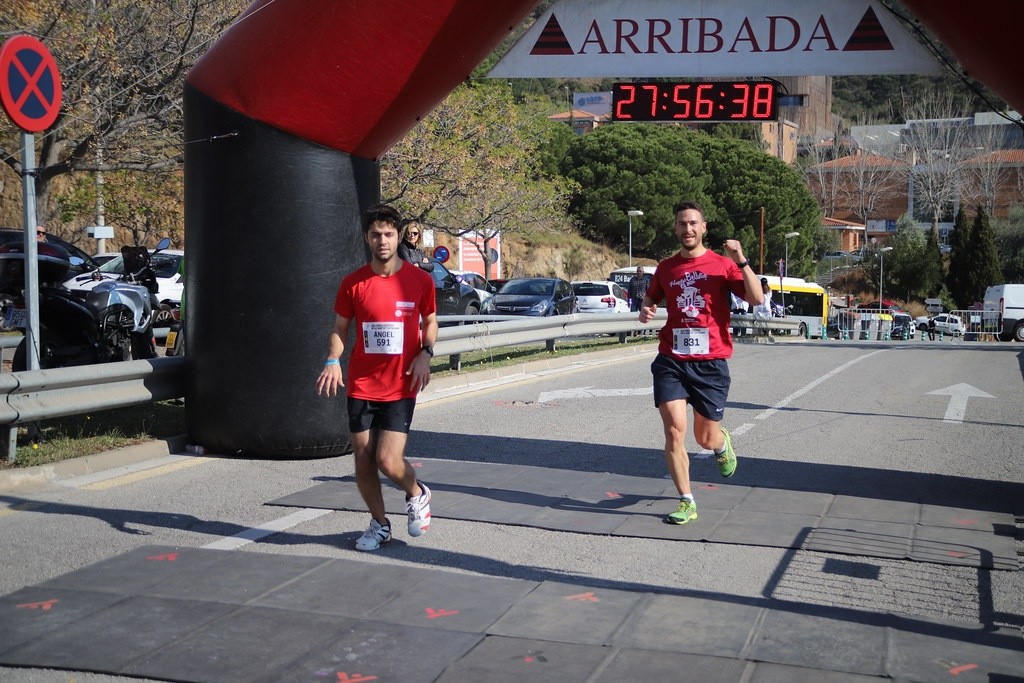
0;227;100;312
916;313;964;336
827;301;917;339
938;242;953;254
489;279;510;291
59;249;184;322
820;250;864;265
482;277;576;319
428;258;481;326
850;249;878;261
570;281;633;312
88;253;119;268
450;271;497;311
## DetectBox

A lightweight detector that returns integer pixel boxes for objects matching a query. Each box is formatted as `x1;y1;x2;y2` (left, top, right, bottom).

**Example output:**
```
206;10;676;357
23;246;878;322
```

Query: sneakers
404;479;432;538
355;517;392;551
666;496;698;525
713;426;737;479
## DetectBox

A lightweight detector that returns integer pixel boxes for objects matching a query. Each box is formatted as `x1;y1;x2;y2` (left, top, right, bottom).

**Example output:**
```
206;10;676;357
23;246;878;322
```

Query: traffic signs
925;299;943;313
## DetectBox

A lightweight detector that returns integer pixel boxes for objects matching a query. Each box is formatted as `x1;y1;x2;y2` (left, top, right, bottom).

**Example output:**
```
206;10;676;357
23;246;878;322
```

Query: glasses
407;231;420;235
36;229;48;235
675;202;700;215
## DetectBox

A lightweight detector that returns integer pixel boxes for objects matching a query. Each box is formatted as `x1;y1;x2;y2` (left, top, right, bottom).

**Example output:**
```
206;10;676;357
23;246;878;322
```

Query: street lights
627;210;643;267
880;247;893;315
784;231;800;277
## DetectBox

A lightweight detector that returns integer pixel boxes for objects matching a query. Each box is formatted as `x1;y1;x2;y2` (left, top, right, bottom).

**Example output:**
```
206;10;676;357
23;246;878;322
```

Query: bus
608;266;828;338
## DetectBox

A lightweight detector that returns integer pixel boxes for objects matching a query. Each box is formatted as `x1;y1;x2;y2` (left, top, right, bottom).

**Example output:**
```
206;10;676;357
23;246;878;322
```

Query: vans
983;284;1024;342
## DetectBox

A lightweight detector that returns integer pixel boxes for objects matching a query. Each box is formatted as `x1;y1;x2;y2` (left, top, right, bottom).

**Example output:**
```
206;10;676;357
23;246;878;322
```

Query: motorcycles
2;237;184;372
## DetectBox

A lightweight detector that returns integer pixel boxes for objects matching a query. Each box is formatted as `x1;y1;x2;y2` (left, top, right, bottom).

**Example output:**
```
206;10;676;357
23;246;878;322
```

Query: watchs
421;345;433;357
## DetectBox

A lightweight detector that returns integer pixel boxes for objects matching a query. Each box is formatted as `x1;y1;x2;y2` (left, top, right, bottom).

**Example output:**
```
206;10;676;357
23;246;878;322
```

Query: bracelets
324;359;340;367
736;257;750;268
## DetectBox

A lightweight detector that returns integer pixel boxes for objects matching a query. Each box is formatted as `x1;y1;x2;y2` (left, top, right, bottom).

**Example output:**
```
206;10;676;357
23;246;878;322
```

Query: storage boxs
0;241;71;295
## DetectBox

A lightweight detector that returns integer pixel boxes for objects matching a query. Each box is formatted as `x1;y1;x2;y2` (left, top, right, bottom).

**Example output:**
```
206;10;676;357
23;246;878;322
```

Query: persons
627;266;649;312
397;221;434;273
37;224;46;242
928;317;938;341
314;206;439;550
639;202;764;524
755;277;772;336
974;299;982;309
731;292;749;336
